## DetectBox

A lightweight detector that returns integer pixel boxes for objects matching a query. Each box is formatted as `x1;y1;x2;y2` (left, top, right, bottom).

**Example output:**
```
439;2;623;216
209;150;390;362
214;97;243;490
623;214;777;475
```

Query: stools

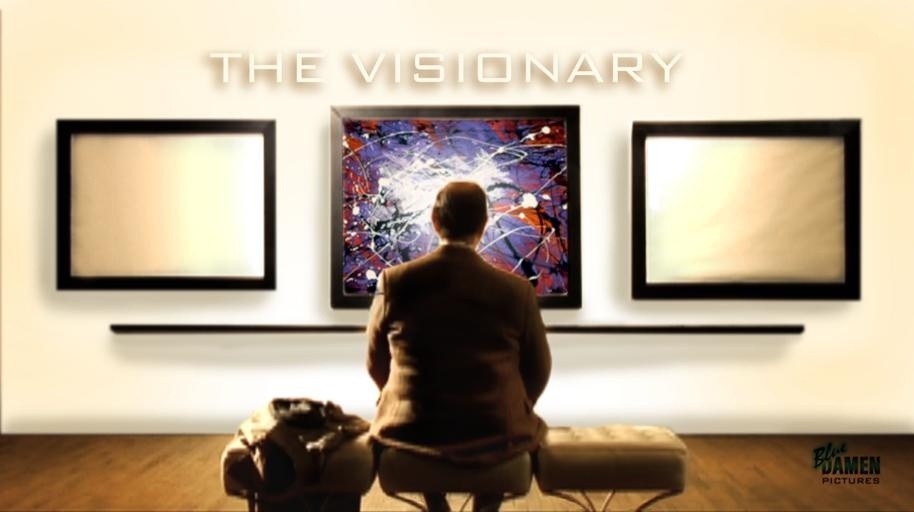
378;449;534;511
534;425;688;512
220;436;375;512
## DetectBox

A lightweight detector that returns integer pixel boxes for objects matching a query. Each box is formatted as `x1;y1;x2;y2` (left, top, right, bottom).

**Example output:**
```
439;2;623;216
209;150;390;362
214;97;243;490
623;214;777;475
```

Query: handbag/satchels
236;397;370;483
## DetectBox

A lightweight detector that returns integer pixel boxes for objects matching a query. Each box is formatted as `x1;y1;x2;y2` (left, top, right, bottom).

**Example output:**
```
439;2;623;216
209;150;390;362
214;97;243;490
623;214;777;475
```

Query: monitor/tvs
328;104;583;311
56;119;276;291
632;119;862;300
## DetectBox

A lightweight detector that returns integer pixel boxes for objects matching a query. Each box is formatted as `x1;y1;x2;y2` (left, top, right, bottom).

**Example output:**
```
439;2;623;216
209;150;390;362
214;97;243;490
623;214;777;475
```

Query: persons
364;178;551;511
